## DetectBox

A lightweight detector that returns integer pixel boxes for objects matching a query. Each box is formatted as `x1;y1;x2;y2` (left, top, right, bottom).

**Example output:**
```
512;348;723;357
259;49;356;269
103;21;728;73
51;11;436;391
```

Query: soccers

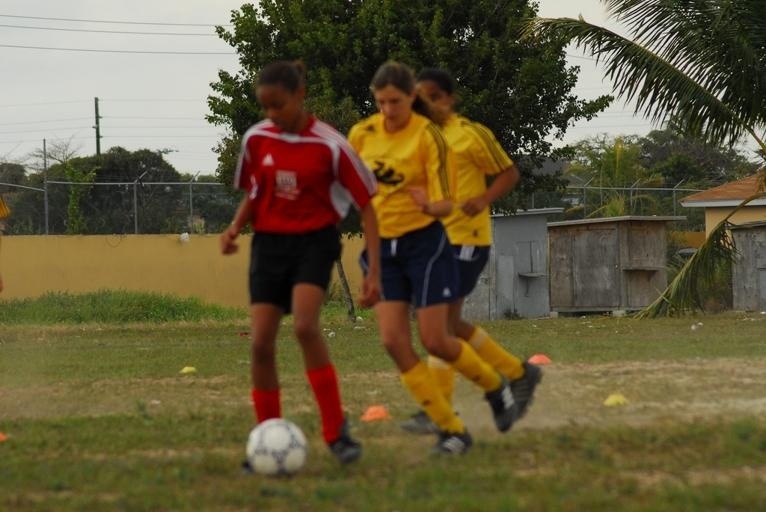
246;418;308;477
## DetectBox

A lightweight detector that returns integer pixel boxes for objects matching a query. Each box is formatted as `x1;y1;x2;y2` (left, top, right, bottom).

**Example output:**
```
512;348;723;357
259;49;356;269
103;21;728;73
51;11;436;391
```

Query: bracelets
230;220;244;234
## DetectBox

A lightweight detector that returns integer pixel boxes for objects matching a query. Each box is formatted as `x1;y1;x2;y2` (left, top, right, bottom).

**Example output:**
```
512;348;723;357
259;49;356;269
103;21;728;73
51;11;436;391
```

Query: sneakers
401;410;472;459
483;360;542;433
328;434;361;464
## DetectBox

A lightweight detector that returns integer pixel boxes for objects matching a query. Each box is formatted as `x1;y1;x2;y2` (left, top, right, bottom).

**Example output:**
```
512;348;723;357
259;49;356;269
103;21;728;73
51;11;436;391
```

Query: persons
345;59;543;459
218;60;383;471
